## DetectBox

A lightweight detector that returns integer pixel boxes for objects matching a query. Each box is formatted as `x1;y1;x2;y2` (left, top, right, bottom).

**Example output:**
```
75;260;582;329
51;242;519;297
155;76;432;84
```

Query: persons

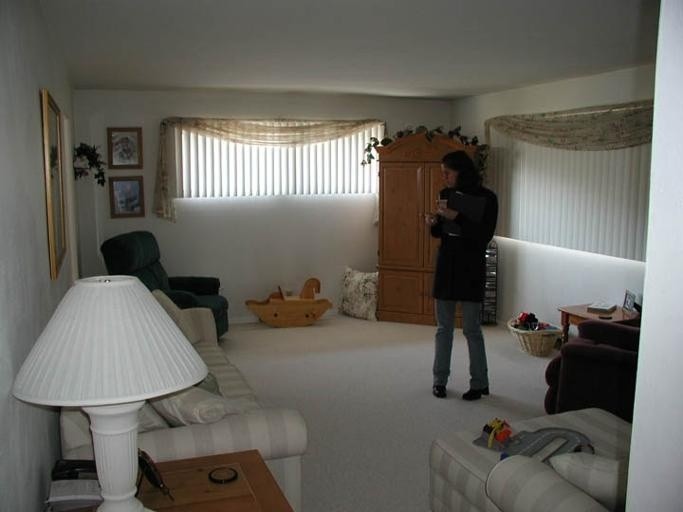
120;181;138;212
117;137;131;159
423;149;501;403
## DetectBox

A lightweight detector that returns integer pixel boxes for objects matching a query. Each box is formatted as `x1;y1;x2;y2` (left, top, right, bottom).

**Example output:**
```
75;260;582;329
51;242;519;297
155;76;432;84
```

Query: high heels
432;383;447;397
463;385;489;400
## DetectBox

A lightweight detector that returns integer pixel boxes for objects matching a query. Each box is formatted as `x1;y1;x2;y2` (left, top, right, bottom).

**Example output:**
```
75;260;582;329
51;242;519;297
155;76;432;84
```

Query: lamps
10;274;209;511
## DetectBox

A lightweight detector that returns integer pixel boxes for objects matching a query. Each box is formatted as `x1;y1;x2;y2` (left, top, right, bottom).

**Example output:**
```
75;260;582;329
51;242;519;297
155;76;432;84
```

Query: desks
42;446;296;512
557;301;641;346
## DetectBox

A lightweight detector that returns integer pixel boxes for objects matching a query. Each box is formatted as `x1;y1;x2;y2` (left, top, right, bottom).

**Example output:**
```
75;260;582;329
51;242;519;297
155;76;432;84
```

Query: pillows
194;370;224;397
548;452;630;512
149;386;244;426
138;400;170;431
149;289;202;345
338;266;383;322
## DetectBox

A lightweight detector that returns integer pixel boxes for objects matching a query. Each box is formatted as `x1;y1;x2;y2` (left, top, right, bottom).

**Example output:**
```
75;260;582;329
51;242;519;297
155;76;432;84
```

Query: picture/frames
621;289;642;318
109;176;145;219
106;125;144;170
40;88;68;283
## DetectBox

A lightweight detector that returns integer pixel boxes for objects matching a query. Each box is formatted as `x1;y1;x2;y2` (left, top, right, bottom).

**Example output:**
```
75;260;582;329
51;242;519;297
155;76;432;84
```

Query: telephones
51;459;99;481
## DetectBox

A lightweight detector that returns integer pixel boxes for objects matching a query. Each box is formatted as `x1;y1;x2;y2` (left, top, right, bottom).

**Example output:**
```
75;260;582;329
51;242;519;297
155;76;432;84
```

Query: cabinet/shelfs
378;269;466;329
378;160;456;271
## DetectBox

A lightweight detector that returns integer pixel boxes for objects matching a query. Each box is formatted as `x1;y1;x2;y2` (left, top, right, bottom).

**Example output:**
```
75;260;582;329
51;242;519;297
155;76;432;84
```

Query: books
587;298;618;313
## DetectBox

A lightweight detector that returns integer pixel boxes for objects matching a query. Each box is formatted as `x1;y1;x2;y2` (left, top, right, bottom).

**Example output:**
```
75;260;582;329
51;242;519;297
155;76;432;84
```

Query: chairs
100;230;229;339
426;407;632;512
543;318;641;425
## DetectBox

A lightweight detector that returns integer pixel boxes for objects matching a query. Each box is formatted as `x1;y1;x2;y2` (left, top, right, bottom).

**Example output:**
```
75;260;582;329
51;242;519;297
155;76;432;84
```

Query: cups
435;199;446;214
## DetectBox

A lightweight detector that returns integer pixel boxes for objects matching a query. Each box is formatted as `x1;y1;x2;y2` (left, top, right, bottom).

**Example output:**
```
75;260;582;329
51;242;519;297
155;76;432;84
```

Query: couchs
55;288;307;512
